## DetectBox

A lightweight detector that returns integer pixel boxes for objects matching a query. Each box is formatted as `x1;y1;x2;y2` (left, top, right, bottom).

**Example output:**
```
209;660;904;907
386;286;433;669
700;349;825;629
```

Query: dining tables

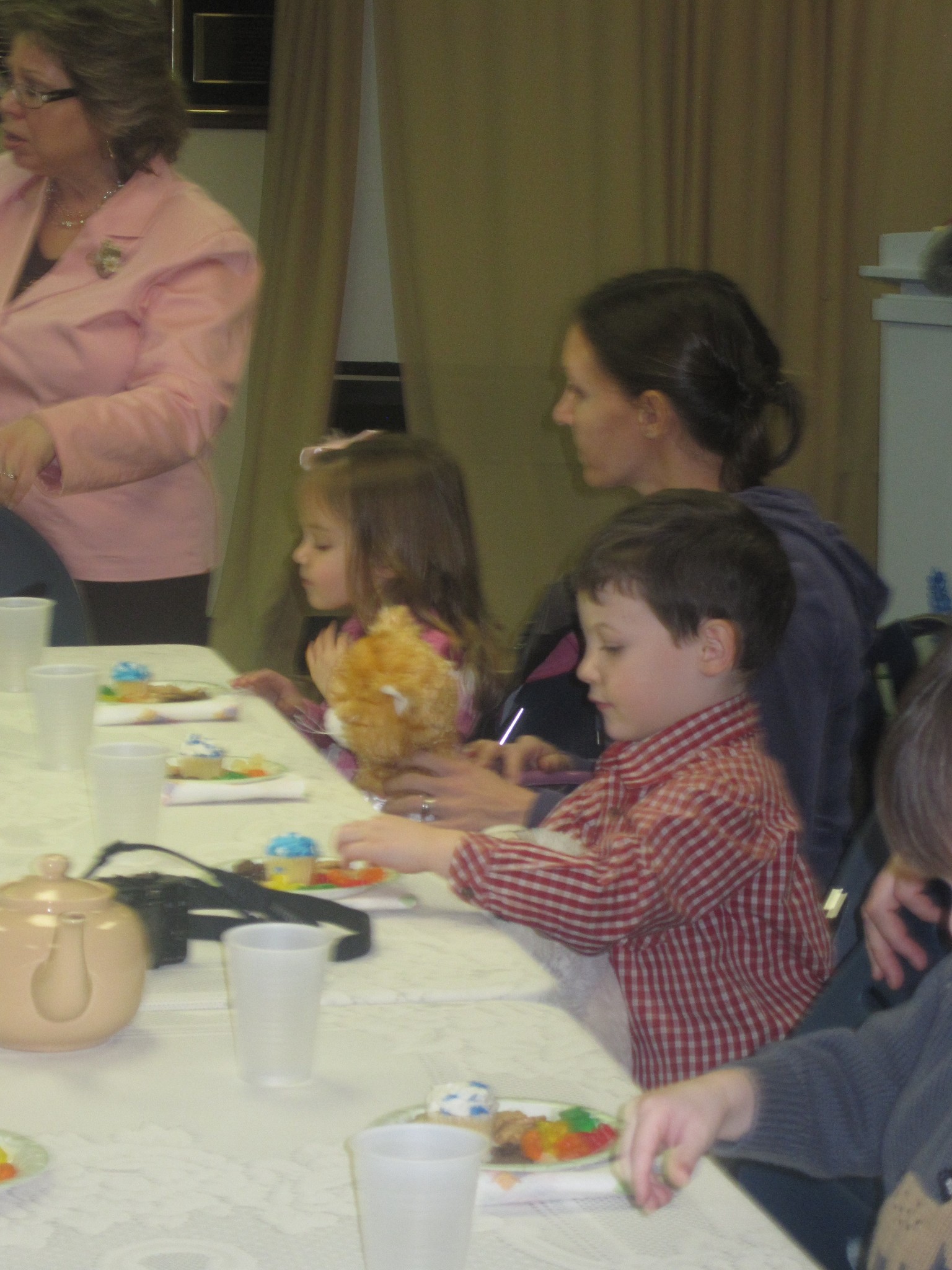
2;642;829;1270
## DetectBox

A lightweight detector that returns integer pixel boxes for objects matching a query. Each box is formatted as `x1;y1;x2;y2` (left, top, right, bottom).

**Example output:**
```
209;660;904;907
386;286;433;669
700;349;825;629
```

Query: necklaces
48;180;131;227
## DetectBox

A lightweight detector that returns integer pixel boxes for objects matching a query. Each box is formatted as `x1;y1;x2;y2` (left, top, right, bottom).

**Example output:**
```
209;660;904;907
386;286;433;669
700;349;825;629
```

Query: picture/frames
165;0;272;129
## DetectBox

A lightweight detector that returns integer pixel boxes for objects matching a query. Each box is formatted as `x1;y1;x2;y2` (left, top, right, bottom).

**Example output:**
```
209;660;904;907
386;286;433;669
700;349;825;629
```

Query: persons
859;843;951;991
228;428;515;793
614;637;951;1269
377;266;900;900
0;0;267;648
334;488;836;1093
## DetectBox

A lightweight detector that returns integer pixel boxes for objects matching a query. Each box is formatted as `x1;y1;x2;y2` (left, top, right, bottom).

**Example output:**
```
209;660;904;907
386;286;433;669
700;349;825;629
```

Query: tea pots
1;850;148;1052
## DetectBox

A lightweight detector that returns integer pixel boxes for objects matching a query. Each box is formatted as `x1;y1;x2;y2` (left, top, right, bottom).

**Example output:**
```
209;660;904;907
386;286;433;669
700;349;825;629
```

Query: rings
421;796;431;821
2;472;16;481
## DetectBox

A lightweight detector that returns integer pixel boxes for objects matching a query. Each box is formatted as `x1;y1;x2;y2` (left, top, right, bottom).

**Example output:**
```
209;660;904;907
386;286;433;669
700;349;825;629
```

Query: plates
380;1098;625;1173
0;1128;49;1193
205;854;398;900
92;680;229;704
160;755;287;784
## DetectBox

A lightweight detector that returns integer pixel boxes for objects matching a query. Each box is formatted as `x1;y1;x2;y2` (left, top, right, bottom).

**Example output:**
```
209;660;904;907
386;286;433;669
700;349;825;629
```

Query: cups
81;739;167;851
220;920;334;1090
23;663;102;770
0;596;50;695
348;1119;492;1270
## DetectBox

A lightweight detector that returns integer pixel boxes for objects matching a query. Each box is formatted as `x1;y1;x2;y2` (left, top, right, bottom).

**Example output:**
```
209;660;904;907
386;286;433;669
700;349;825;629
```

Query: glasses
0;70;80;109
